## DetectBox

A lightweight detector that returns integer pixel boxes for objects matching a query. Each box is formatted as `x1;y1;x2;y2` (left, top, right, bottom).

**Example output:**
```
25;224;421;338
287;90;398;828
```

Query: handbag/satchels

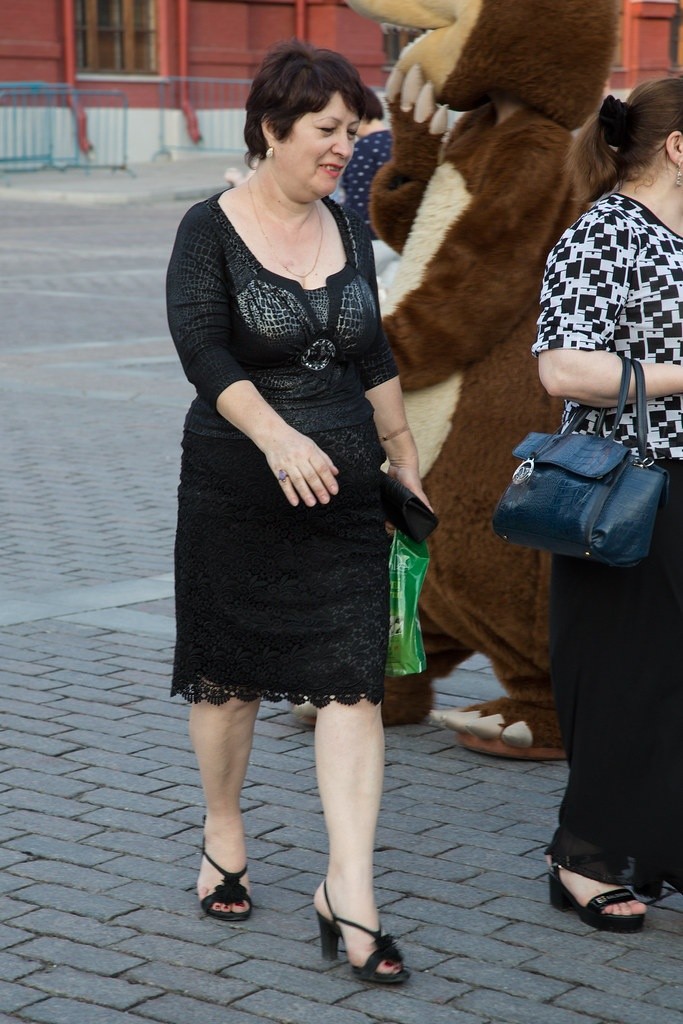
492;354;670;568
385;531;431;676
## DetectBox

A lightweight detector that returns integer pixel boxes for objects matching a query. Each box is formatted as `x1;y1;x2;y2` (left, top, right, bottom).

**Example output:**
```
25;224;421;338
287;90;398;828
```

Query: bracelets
381;427;409;443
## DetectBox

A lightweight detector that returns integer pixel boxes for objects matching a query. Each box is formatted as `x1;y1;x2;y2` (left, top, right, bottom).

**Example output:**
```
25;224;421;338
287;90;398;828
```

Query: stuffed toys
289;2;620;761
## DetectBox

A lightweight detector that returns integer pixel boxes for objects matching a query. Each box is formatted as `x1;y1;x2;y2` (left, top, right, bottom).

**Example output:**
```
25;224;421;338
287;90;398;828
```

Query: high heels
316;879;411;984
635;857;683;898
199;815;253;922
544;829;645;933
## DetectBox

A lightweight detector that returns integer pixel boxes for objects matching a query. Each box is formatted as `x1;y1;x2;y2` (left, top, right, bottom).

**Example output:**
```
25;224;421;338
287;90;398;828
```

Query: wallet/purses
379;475;439;544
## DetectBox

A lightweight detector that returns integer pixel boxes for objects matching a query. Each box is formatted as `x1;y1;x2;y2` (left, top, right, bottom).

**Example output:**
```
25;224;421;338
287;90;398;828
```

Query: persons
531;74;683;935
162;36;437;988
342;78;393;240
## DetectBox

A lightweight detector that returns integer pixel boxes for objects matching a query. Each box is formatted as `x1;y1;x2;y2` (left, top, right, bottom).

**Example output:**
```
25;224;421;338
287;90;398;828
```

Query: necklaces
246;183;323;277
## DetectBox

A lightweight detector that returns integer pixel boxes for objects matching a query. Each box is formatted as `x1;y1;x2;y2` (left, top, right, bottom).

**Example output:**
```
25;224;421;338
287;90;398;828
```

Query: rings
277;469;289;481
